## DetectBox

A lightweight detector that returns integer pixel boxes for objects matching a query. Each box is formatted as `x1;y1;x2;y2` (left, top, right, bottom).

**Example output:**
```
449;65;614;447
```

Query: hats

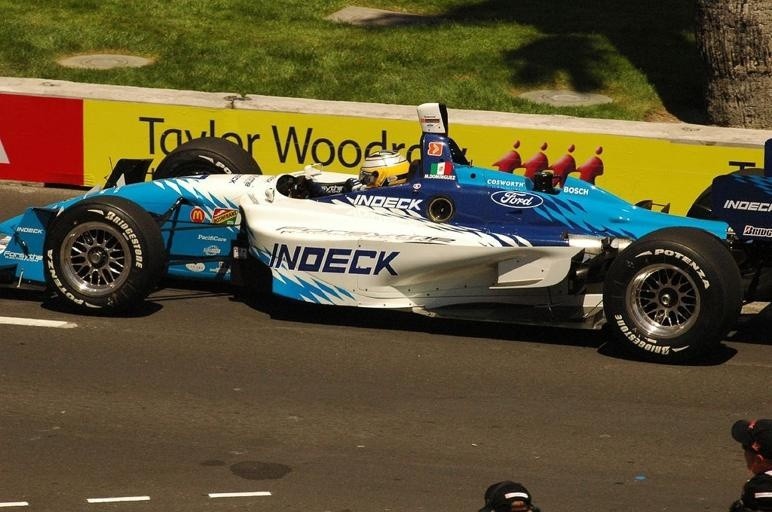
483;481;532;512
730;416;772;458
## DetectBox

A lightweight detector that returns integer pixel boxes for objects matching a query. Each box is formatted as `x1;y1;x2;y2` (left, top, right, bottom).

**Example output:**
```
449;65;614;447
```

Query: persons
727;416;772;512
477;481;541;512
289;147;411;200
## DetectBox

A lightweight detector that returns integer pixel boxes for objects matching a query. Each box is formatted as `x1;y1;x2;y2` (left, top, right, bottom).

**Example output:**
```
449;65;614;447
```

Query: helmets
358;150;411;189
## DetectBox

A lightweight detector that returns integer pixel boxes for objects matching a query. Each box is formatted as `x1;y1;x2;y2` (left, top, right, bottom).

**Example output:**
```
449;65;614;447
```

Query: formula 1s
0;104;740;365
